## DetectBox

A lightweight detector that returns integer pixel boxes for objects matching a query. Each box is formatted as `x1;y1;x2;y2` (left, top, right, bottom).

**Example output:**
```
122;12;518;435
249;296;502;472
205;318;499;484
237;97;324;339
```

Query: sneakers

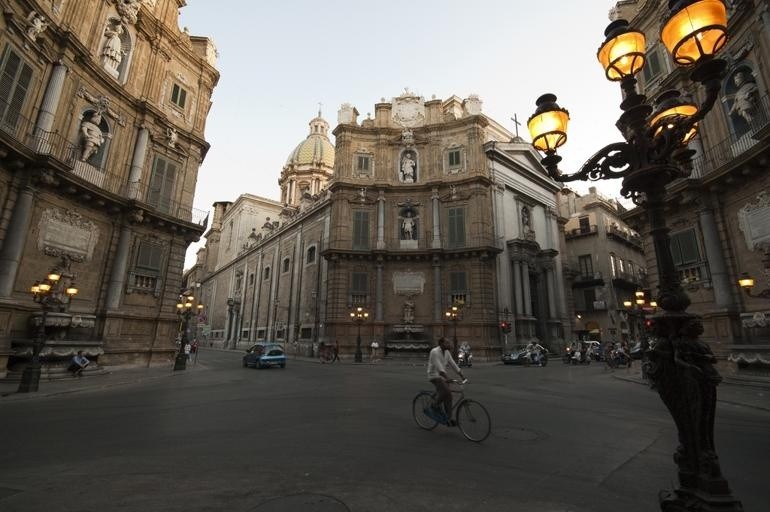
448;419;457;427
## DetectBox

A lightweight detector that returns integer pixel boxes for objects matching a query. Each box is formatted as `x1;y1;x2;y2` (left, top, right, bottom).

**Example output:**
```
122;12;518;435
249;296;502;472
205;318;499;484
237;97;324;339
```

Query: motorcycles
522;344;547;367
457;345;473;369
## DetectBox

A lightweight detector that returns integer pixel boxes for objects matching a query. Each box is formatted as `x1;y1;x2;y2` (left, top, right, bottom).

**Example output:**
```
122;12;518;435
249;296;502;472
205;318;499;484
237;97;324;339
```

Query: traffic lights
646;319;656;336
507;322;512;333
502;322;507;334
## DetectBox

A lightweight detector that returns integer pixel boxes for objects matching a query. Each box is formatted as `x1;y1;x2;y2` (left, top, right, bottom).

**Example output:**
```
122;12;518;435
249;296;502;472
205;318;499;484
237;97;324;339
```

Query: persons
80;112;105;161
190;336;198;354
426;337;467;427
724;72;758;126
24;15;48;51
184;341;191;365
401;153;415;180
461;342;471;365
331;340;340;363
563;337;611;367
102;24;125;71
369;339;379;362
402;211;415;239
528;340;539;361
68;350;90;374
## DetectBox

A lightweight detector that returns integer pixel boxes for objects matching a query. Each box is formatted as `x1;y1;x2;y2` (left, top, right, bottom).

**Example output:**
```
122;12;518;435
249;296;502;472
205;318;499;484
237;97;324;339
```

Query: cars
501;343;548;365
562;338;657;368
176;333;198;354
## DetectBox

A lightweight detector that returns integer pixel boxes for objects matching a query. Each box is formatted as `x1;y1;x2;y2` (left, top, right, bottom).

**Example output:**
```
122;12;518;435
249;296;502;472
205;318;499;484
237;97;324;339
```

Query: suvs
242;342;286;370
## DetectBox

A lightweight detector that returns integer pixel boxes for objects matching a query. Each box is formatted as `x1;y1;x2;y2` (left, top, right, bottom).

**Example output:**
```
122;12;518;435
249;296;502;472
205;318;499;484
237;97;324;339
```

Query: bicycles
412;377;492;443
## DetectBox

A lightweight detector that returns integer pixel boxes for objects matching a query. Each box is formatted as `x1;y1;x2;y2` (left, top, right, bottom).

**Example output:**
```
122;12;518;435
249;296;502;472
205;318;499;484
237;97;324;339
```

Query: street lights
623;287;658;380
737;269;770;300
350;307;369;363
527;1;744;511
17;265;78;393
174;292;204;370
445;305;464;360
